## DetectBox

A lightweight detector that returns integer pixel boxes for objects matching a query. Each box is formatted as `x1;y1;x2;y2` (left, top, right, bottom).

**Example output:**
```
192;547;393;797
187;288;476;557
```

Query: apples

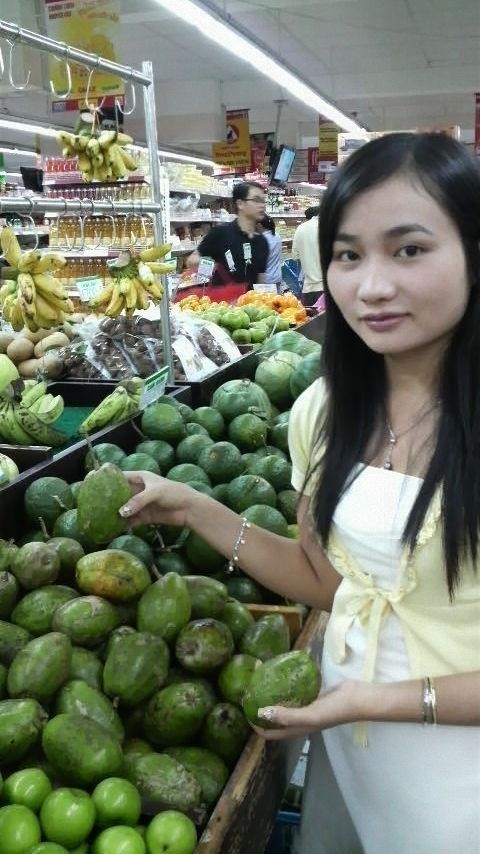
179;289;307;344
0;768;198;854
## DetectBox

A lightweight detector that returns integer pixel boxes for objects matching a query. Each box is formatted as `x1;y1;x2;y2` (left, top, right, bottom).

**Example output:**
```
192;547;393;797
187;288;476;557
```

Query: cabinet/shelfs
0;112;330;350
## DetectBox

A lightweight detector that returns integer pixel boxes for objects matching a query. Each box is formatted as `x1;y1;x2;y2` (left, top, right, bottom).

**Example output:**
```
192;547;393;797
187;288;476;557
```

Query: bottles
263;186;300;214
50;156;155;288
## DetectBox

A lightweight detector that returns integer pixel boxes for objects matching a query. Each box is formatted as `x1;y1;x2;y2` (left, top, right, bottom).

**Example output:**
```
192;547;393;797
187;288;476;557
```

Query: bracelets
421;677;437;727
223;516;251;576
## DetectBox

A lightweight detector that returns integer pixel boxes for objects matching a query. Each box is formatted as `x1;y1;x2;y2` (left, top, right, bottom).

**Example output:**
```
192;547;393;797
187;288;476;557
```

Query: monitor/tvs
268;144;297;187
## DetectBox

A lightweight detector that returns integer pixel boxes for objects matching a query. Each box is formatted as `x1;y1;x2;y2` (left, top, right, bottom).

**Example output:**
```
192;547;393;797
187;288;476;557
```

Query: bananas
0;227;176;445
55;126;137;185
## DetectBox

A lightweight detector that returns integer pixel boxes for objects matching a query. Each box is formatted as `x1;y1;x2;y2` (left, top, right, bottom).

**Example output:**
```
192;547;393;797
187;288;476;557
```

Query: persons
120;133;480;854
290;206;326;318
255;215;284;295
184;182;267;293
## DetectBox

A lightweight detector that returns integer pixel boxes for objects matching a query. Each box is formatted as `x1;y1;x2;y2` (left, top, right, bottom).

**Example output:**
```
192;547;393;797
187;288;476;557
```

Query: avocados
0;395;321;825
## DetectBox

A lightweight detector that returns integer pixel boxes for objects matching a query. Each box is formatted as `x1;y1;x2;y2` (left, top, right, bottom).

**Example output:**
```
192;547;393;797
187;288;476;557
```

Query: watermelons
209;330;323;422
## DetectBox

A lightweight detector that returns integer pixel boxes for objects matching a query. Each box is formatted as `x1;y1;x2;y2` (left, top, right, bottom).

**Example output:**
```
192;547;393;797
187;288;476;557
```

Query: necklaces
383;401;442;470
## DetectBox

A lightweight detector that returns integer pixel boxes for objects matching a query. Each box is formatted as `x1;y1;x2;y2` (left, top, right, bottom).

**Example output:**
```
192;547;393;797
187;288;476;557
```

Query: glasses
242;196;267;203
